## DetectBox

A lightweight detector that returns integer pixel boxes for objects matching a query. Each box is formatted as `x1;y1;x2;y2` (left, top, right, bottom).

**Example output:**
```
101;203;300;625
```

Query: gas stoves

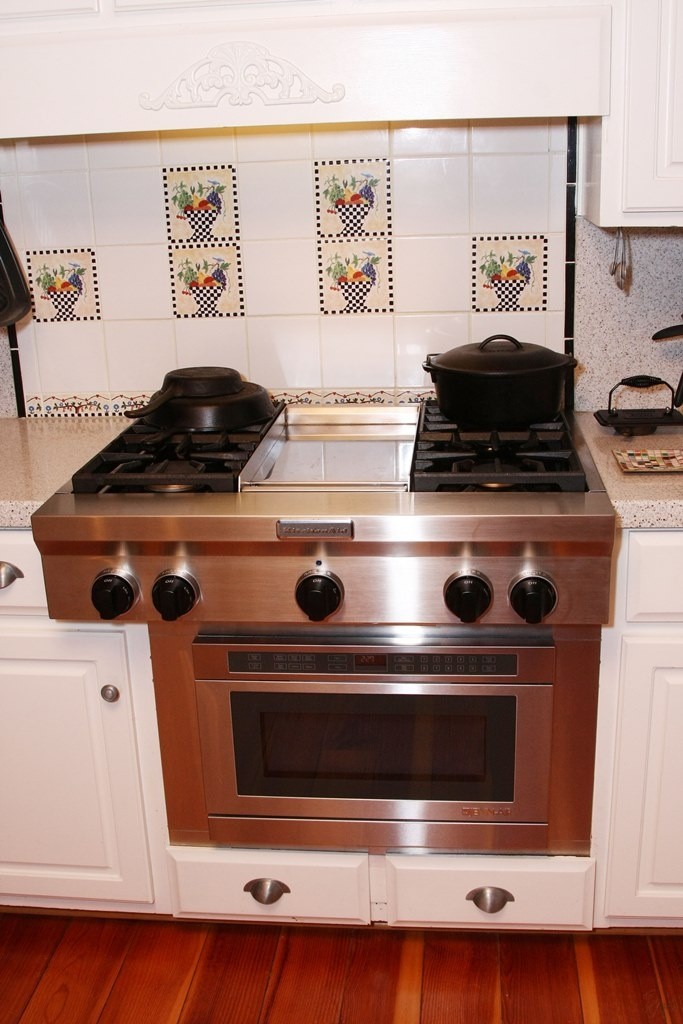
30;410;613;625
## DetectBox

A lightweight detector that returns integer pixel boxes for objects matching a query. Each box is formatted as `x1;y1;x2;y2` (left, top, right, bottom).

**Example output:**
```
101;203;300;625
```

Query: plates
611;449;683;473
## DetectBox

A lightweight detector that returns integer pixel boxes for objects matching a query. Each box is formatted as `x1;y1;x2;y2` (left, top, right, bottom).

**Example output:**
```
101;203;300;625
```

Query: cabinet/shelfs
586;0;683;227
0;530;683;932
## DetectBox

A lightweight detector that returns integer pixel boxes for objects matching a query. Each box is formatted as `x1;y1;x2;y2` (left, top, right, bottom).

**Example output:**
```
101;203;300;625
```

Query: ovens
146;625;602;856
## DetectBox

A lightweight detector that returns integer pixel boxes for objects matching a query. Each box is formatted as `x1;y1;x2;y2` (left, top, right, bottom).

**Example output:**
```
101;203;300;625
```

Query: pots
420;334;577;427
124;369;279;450
